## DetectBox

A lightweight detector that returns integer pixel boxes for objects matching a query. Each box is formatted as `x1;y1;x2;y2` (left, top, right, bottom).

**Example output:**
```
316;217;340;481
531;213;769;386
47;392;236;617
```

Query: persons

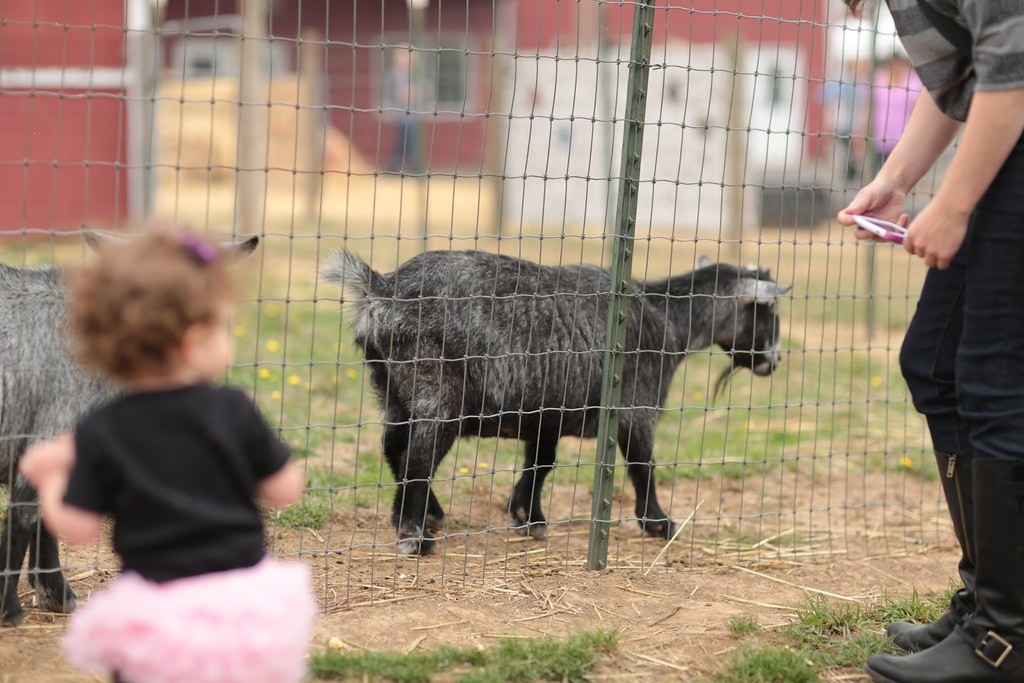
388;43;421;177
18;225;321;683
838;0;1024;682
852;50;922;173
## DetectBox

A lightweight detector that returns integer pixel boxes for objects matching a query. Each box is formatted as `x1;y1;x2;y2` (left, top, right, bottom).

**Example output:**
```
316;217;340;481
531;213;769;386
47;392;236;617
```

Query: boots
865;457;1024;683
886;446;979;654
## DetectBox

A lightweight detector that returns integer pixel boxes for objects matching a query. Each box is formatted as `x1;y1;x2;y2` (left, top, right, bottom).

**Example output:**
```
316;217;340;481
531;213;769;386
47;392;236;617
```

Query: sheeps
323;243;789;570
0;232;265;625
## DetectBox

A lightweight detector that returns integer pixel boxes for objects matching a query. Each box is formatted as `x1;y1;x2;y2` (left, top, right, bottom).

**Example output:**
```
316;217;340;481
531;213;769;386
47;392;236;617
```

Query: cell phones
853;214;908;244
